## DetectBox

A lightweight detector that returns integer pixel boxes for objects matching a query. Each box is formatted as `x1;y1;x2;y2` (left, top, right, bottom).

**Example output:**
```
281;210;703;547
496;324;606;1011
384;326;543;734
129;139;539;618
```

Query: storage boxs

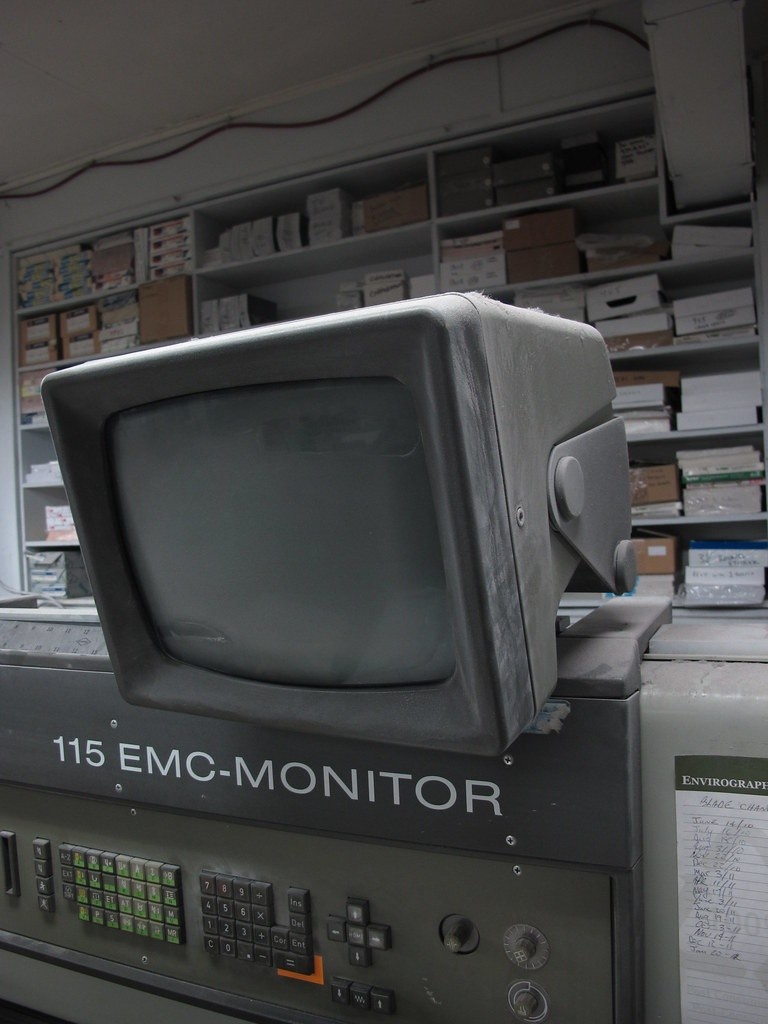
20;135;768;609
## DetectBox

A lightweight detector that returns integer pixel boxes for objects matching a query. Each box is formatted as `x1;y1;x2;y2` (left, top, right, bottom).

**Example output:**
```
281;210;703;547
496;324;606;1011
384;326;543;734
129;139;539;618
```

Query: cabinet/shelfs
0;89;768;619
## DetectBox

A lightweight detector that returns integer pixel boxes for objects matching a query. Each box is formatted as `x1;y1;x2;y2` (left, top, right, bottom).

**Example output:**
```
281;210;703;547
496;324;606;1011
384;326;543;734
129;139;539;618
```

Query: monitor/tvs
36;292;634;759
